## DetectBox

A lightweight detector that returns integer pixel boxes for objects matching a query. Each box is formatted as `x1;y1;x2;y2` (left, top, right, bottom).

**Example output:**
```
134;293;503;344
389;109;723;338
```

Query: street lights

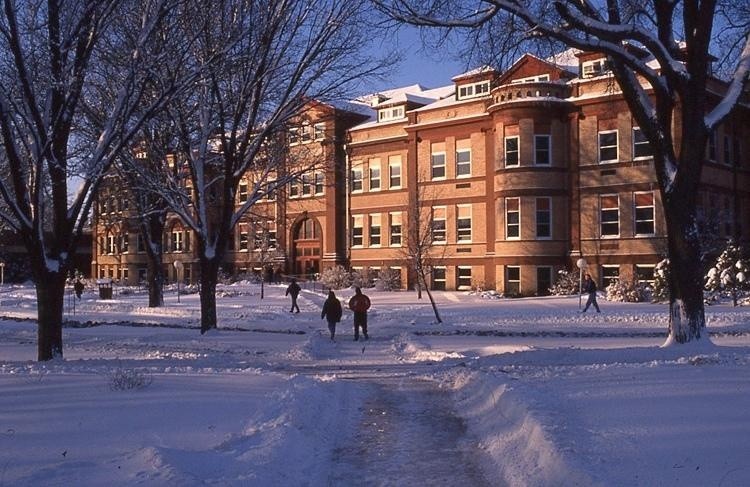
573;255;589;312
172;258;187;305
1;261;9;286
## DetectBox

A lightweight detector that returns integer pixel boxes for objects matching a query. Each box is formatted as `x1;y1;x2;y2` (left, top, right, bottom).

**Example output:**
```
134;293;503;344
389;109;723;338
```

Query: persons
322;291;342;340
582;274;600;312
349;287;371;342
285;279;301;313
74;279;84;301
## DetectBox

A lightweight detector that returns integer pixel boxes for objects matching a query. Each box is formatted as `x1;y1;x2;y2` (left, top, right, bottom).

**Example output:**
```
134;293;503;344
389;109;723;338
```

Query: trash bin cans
96;278;112;299
